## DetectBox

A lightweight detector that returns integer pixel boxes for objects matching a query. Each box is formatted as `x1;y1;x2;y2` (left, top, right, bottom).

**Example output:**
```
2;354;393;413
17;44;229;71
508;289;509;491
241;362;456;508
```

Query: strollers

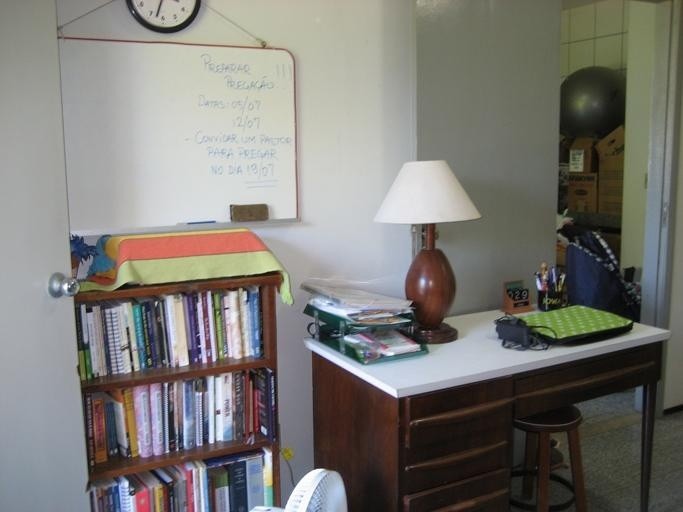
565;230;641;322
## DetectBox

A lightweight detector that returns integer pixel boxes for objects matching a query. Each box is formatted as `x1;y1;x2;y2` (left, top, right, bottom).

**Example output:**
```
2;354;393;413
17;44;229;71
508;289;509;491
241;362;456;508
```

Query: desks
302;304;672;511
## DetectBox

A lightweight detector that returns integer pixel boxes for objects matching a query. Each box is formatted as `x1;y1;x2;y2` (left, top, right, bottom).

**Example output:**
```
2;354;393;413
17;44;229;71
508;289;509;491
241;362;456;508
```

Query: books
91;445;274;511
75;285;264;379
83;366;275;468
300;281;420;361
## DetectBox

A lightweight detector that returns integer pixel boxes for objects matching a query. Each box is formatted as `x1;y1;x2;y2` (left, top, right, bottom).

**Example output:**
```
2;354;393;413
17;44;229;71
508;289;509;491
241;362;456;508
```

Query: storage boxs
559;126;624;216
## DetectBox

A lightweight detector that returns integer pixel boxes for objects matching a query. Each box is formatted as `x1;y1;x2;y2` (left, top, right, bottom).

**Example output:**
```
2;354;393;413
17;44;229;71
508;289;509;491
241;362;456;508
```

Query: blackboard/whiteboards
59;37;299;240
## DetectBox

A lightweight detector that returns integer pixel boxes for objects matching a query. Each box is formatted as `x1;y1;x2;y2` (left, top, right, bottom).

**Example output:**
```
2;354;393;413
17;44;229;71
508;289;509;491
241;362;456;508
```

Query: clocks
124;1;200;33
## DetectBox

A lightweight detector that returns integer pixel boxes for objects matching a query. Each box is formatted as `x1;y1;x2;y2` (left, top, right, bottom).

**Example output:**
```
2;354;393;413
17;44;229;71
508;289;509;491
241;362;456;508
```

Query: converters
496;323;529;346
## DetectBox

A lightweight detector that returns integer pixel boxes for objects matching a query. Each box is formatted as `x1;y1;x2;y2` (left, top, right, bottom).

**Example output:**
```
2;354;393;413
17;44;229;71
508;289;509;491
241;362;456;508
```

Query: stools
509;404;586;511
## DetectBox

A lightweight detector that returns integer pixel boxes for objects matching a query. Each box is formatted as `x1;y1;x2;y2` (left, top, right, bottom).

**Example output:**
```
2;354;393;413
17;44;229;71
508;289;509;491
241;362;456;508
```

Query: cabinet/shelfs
398;339;664;511
75;273;283;511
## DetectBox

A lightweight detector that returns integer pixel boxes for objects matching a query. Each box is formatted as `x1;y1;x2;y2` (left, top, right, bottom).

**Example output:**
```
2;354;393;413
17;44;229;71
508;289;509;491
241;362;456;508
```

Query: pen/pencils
534;263;567;292
187;221;215;224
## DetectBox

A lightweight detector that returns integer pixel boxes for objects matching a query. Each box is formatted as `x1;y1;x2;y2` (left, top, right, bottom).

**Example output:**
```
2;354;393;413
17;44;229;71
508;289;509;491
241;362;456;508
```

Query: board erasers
230;205;268;220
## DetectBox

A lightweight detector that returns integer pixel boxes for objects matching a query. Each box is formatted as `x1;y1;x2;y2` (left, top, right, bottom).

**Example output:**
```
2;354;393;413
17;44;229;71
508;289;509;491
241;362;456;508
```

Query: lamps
372;159;483;343
